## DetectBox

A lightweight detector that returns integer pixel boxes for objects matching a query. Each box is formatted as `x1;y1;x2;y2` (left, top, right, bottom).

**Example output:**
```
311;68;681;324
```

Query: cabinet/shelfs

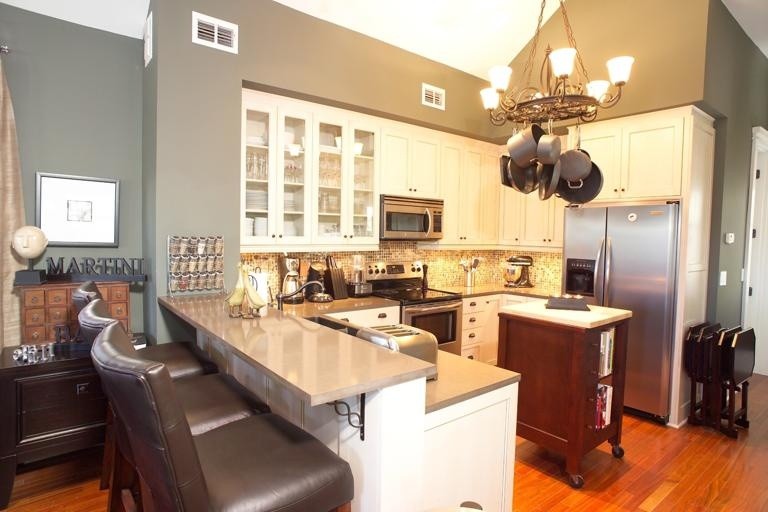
328;307;401;333
575;107;715;201
459;294;498;364
311;104;380;251
380;121;438;201
500;142;522;250
439;130;500;248
498;301;632;485
521;123;573;251
241;90;312;253
20;282;129;347
0;332;149;512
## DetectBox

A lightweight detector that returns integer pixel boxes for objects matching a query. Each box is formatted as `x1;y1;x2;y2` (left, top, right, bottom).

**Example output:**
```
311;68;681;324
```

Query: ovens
400;300;462;357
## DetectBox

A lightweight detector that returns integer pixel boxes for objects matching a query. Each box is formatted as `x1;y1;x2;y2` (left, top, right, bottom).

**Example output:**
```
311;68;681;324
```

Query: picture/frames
34;172;121;248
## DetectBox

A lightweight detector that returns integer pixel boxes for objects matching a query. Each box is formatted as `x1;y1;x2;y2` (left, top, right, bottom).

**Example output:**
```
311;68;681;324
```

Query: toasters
353;323;438;382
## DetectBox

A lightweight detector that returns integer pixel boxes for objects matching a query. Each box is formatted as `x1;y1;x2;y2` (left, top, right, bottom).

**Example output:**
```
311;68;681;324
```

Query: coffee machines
279;257;304;304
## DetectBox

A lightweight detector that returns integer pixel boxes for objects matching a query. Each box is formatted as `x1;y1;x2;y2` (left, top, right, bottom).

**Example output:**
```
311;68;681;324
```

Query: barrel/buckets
463;270;476;288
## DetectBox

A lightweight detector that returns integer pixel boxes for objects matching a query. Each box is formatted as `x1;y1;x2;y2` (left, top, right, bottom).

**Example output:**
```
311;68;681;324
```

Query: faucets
276;280;326;311
276;311;324;333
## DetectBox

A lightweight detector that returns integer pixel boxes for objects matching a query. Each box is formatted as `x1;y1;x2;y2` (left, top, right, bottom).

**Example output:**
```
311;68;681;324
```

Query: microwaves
378;193;444;240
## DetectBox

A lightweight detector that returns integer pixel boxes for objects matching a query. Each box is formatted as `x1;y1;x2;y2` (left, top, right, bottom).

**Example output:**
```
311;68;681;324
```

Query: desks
153;290;439;512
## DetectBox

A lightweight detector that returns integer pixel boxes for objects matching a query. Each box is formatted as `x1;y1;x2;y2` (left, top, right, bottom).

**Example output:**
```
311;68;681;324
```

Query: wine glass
284;159;367;191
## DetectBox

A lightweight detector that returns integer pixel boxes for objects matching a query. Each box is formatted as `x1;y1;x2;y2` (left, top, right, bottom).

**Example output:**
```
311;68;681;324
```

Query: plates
247;190;269;210
247;216;297;237
284;199;297;211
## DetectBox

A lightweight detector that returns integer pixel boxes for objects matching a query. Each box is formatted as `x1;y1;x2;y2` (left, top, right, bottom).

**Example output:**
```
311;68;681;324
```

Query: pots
499;117;604;208
346;281;373;297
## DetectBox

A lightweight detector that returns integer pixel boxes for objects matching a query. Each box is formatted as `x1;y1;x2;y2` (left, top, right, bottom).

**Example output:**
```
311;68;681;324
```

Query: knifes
327;255;338;269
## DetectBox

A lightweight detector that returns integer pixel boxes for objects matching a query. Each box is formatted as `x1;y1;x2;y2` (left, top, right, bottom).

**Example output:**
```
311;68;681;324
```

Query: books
595;327;616;431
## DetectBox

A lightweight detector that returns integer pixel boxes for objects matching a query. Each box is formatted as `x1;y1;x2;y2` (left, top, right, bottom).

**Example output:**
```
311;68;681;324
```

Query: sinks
337;327;360;337
304;316;348;330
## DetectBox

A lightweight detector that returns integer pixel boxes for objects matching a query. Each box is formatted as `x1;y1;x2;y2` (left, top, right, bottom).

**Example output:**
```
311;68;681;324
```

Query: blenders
502;256;535;288
306;261;334;302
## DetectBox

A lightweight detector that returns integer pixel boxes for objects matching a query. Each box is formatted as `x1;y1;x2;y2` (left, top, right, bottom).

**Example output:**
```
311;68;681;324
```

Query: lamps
505;255;534;287
480;1;635;125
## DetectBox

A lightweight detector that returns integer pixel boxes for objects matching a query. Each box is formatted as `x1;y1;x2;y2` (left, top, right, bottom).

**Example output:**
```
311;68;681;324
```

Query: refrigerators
563;200;677;426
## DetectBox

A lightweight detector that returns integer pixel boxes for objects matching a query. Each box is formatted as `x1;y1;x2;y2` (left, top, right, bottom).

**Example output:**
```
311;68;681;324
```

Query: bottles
422;265;429;292
320;191;329;213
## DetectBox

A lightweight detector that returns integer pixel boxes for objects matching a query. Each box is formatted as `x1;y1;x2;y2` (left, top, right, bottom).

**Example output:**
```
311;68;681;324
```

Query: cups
28;345;38;363
463;271;475;287
318;223;366;237
247;152;267;181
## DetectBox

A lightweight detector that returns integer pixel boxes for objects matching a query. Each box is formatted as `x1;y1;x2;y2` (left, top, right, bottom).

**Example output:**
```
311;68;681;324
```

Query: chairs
69;278;220;490
87;323;355;512
74;298;271;511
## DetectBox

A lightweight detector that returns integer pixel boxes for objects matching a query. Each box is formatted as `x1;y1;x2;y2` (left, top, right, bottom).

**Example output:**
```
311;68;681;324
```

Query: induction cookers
366;277;462;305
